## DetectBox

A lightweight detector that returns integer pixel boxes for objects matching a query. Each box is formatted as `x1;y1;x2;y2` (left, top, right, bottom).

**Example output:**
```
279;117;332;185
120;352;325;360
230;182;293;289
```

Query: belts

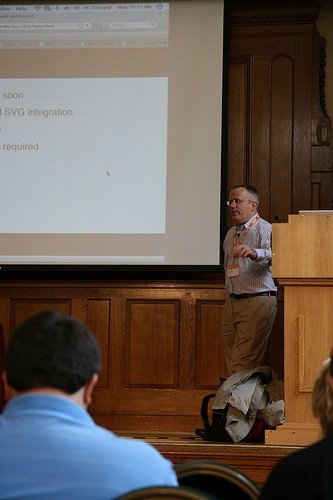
229;291;277;299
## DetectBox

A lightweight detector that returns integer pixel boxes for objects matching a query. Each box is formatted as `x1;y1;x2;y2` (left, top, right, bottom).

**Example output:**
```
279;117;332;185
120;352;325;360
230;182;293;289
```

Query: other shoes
195;428;206;436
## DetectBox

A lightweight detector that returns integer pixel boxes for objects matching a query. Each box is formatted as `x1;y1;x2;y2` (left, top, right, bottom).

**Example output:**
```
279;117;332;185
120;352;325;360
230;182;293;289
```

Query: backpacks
201;387;269;443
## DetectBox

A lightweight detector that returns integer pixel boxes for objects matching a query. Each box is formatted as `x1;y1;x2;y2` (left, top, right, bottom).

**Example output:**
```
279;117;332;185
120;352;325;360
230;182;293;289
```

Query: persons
258;359;333;500
221;185;277;376
0;309;179;500
